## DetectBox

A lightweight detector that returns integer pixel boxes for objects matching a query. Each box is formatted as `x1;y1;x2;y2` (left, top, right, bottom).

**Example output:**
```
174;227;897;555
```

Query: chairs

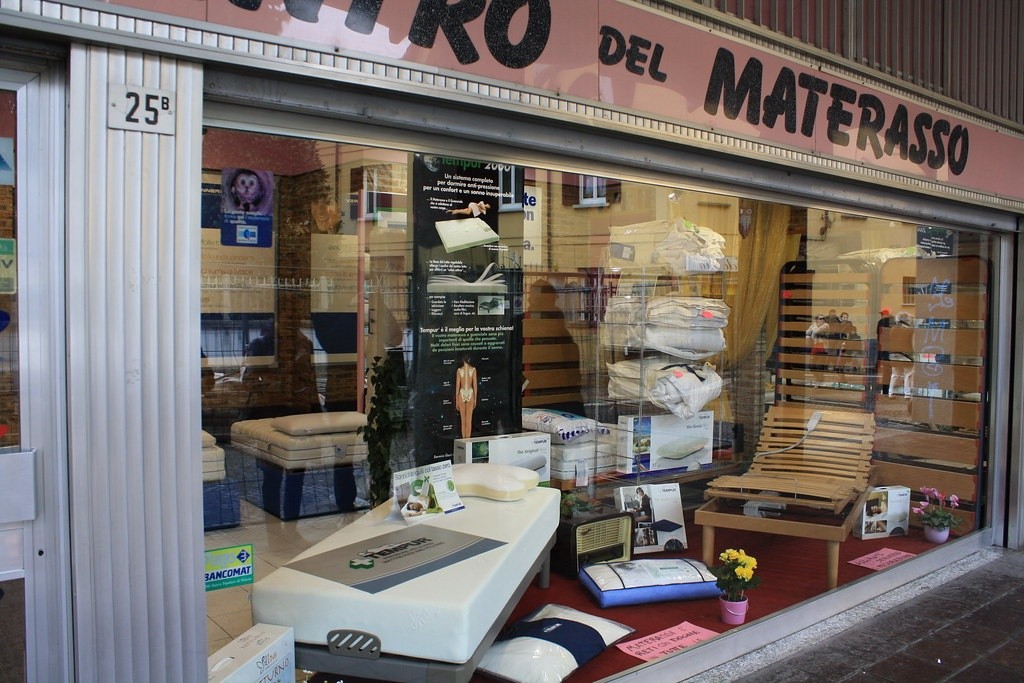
694;405;877;593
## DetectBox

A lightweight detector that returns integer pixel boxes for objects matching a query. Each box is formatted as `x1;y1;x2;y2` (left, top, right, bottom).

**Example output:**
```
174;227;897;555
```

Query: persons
805;309;861;388
626;487;651;528
876;310;914;400
242;301;318;549
456;352;477;439
868;495;888;517
201;347;215;394
446;201;490;217
404;497;429;517
314;266;403;526
505;280;586;419
310;196;337;235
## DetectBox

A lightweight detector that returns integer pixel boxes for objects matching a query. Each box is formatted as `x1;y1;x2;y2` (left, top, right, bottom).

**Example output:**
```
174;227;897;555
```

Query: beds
249;484;561;683
530;423;622;490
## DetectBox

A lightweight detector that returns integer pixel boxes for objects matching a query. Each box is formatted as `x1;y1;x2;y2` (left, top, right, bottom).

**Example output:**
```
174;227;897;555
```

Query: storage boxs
454;431;551;489
208;624;295;683
612;481;688;554
618;411;715;475
853;485;911;540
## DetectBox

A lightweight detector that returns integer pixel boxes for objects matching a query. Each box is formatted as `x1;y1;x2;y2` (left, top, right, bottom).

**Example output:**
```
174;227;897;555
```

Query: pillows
202;430;220;447
273;412;366;436
522;407;610;444
450;463;540;501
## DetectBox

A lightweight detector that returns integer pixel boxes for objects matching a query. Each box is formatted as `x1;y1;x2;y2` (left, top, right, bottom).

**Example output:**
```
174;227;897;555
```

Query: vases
719;596;748;625
924;525;950;544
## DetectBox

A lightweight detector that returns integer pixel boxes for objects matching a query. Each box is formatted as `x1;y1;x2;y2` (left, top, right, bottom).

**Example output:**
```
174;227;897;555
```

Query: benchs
230;415;368;520
202;427;241;531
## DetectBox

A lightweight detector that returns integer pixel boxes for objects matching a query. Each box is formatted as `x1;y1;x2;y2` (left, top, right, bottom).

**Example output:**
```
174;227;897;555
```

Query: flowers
913;484;963;531
714;547;758;600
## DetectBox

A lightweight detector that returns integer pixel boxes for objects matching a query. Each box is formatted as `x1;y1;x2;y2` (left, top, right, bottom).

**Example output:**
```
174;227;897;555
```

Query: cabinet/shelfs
592;275;739;500
